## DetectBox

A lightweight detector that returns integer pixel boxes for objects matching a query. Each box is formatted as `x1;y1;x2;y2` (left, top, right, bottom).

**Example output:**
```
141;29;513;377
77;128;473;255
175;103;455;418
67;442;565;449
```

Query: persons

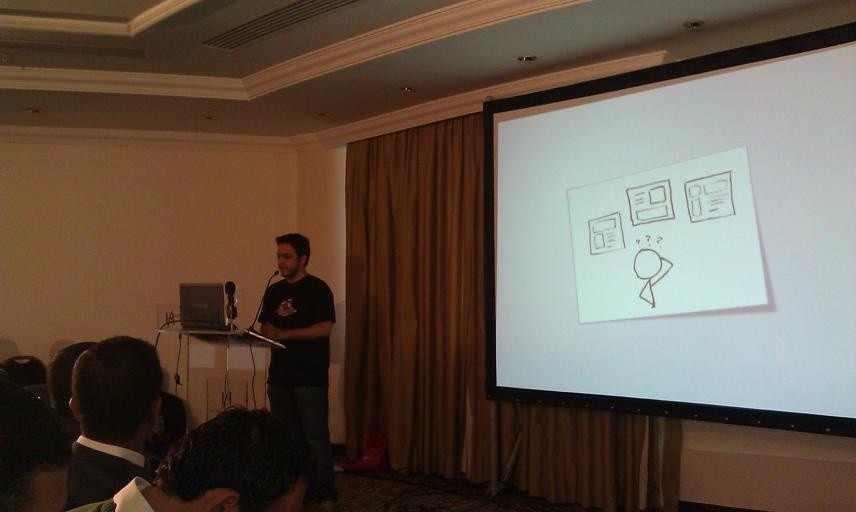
144;388;190;478
257;232;342;512
0;372;82;512
47;338;98;481
65;403;319;512
59;334;167;507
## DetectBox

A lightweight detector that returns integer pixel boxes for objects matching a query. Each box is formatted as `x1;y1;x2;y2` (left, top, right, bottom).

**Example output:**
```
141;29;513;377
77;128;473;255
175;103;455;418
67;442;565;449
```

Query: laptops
180;282;238;331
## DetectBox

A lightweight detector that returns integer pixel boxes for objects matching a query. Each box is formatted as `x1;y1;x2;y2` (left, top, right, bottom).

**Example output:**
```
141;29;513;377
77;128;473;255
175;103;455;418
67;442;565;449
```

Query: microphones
225;281;237;319
246;270;279;336
